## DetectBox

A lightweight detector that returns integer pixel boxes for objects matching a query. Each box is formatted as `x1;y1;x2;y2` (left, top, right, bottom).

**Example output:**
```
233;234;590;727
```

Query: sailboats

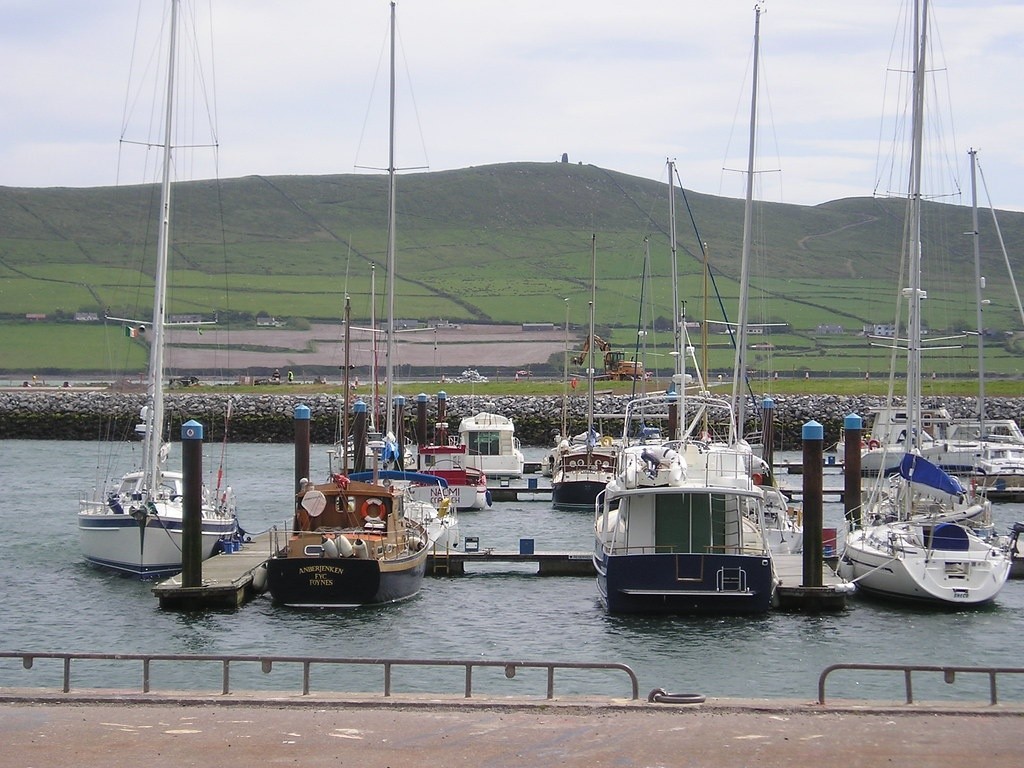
78;1;243;580
261;0;1023;614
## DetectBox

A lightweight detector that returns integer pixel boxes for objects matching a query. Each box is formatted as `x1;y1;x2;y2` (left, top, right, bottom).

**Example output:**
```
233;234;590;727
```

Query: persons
760;464;772;486
571;377;576;390
272;367;280;379
288;368;294;381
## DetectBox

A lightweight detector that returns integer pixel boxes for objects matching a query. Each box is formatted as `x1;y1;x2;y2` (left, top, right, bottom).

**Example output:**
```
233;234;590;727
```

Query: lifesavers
360;498;385;523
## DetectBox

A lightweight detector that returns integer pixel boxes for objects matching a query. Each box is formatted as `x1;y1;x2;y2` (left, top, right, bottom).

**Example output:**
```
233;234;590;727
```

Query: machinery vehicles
571;333;643;383
